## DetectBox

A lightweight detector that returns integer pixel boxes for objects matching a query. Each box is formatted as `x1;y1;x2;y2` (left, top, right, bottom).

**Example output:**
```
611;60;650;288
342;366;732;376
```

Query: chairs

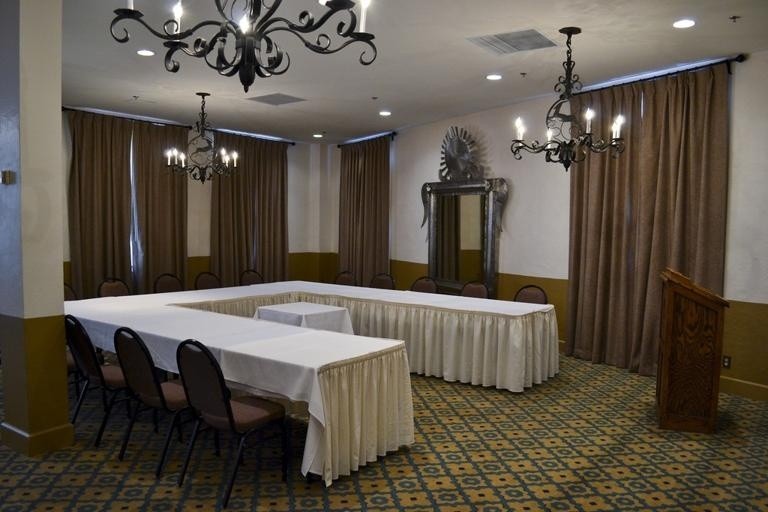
194;271;223;291
64;281;105;377
112;326;191;479
172;335;291;510
513;283;548;306
97;277;132;297
461;280;490;299
410;276;439;294
370;273;396;290
334;271;354;287
153;273;185;293
238;269;265;286
65;314;125;451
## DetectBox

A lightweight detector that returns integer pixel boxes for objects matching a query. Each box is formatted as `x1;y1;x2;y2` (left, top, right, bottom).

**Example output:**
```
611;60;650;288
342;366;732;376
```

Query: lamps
165;91;238;183
106;0;378;94
510;24;626;171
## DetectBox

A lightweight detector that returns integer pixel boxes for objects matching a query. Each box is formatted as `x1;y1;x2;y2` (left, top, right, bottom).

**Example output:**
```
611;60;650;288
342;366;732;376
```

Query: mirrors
419;178;509;296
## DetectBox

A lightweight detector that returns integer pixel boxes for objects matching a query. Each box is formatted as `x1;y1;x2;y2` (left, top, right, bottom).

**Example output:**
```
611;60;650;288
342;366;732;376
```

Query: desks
299;279;560;394
64;304;416;493
64;279;302;320
256;299;354;336
